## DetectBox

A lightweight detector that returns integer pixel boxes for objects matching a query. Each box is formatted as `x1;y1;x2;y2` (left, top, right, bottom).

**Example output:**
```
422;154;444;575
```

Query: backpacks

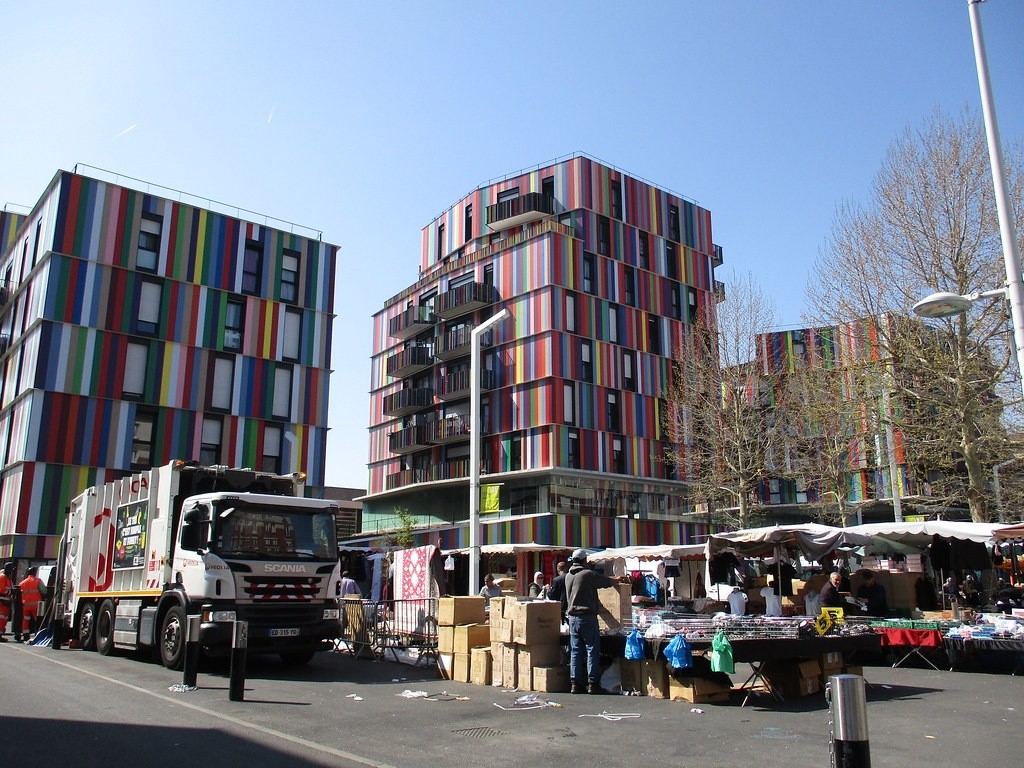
558;573;568;619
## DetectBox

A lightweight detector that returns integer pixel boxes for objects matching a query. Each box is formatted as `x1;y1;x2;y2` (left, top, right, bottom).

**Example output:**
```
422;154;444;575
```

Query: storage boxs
745;554;926;618
763;650;874;696
437;595;571;693
596;583;632;634
619;656;733;704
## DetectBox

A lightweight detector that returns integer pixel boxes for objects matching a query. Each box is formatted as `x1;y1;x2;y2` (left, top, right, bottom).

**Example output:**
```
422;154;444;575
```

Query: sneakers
571;682;587;693
588;682;609;694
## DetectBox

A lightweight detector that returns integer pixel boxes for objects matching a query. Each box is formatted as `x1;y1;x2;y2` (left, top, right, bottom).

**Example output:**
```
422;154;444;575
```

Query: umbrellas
703;521;873;616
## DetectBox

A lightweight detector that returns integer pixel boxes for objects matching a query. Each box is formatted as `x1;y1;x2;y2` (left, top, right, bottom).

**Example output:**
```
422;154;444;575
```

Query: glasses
537;577;543;579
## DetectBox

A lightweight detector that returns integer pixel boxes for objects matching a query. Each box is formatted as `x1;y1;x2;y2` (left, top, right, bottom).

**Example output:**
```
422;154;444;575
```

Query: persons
921;573;939;610
990;576;1017;615
760;581;783;617
942;569;981;607
728;588;748;617
819;572;861;616
658;579;672;606
17;566;48;642
858;571;890;618
478;573;504;606
757;575;768;587
527;570;552;602
563;549;630;694
341;570;362;632
547;562;569;627
805;589;822;617
0;561;20;643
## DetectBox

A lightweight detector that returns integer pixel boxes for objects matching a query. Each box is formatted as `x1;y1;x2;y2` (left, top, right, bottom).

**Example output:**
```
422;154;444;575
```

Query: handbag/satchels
537;586;550;600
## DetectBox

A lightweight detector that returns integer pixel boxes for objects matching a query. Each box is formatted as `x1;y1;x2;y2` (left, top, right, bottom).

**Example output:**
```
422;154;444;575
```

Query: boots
0;635;8;642
24;633;30;641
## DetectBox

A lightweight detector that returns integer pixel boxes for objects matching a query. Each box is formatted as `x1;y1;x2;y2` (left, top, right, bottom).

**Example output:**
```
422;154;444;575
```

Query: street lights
912;286;1023;394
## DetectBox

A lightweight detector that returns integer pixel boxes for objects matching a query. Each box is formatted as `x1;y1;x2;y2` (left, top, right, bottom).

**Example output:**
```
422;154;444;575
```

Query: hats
572;549;589;560
863;571;873;579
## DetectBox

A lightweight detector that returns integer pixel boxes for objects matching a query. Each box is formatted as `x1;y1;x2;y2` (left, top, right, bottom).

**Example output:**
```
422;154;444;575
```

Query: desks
874;627;944;671
943;638;1024;676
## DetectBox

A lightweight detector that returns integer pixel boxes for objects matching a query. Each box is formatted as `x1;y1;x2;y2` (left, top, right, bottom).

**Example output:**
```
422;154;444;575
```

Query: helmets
4;562;16;570
27;567;37;575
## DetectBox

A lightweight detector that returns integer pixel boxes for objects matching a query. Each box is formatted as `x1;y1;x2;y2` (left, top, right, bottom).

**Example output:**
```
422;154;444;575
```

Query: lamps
444;552;459;571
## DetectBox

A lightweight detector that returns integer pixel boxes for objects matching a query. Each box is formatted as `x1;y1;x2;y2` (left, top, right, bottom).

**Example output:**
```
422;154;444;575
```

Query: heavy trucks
61;460;350;671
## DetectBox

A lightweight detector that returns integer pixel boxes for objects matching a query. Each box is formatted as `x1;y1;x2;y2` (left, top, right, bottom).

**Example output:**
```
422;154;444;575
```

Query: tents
586;545;785;612
992;520;1024;586
